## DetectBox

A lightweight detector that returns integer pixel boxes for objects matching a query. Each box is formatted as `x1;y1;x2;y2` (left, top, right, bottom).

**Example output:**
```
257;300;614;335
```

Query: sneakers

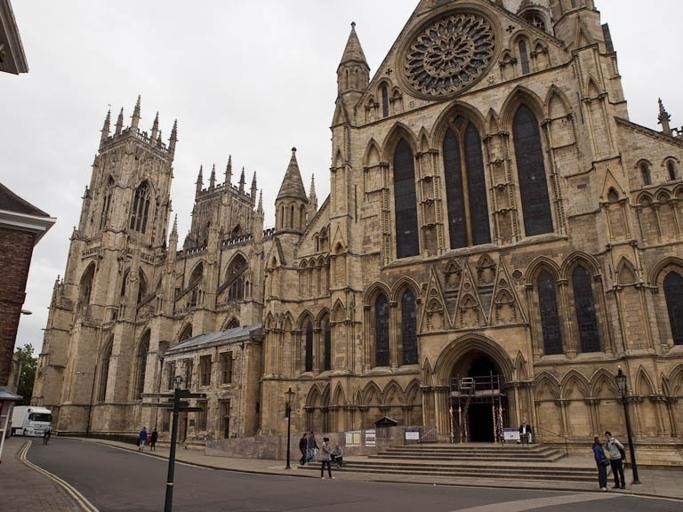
601;487;607;492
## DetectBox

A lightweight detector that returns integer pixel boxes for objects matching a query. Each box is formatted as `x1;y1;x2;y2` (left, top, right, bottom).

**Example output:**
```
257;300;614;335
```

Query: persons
330;441;344;468
516;421;533;444
138;426;148;451
151;427;158;451
591;434;608;492
307;431;319;462
319;438;335;480
146;432;151;446
42;424;53;445
603;431;627;490
298;433;308;465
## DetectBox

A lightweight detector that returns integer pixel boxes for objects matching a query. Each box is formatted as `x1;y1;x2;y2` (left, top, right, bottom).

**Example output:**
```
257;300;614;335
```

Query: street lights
283;388;295;470
613;365;641;485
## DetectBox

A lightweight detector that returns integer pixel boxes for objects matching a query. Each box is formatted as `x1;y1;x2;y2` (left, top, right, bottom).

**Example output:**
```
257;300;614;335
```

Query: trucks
11;405;52;438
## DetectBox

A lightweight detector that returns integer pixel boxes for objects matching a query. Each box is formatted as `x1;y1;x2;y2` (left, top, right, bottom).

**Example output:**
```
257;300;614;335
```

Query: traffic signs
140;402;174;409
140;393;174;398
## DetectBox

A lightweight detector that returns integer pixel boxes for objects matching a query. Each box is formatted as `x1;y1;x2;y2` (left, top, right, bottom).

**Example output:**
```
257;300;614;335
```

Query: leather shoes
613;486;625;489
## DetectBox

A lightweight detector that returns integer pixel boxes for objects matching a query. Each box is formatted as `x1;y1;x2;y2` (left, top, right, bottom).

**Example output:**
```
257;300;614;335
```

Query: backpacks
619;449;625;460
